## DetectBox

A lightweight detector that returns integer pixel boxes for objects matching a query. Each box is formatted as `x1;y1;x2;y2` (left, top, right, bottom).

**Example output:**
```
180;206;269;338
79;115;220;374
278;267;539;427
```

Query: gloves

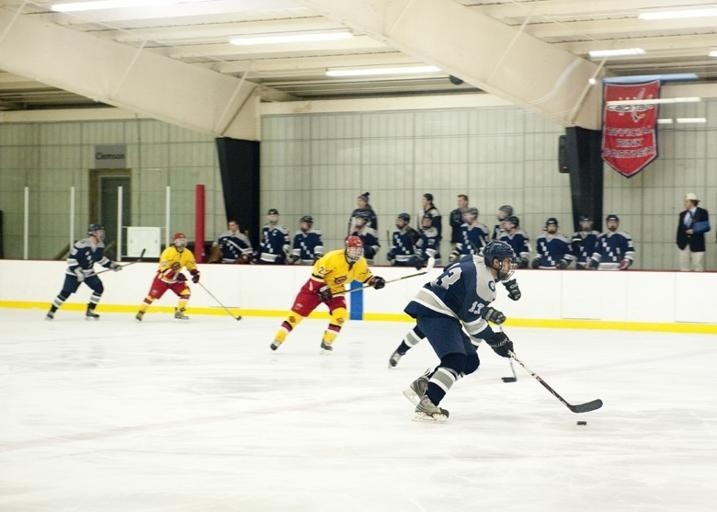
502;279;521;300
177;274;187;281
369;277;385;289
486;332;513;358
319;289;331;301
74;267;85;282
483;308;505;324
192;271;199;283
110;262;122;271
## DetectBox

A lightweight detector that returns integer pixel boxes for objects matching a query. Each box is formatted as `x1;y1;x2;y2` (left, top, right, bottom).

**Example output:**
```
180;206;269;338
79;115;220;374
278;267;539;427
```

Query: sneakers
87;309;99;317
416;396;448;420
410;375;429;399
271;341;279;350
136;312;143;320
175;313;189;319
390;350;401;367
321;343;332;351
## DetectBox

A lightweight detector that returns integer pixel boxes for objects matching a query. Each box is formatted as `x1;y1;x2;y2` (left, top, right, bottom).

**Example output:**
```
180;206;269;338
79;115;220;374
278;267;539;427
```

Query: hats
47;311;54;319
358;192;370;202
685;194;698;200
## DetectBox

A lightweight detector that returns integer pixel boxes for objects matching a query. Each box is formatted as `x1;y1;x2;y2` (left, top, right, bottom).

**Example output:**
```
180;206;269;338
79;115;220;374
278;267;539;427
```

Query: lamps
228;30;351;47
325;65;440;78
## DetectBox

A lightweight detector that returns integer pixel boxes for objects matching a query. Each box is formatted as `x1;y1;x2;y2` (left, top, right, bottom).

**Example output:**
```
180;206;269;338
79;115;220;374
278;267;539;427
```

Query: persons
135;232;200;321
402;238;517;420
388;252;520;369
270;236;386;351
675;193;711;272
46;223;123;319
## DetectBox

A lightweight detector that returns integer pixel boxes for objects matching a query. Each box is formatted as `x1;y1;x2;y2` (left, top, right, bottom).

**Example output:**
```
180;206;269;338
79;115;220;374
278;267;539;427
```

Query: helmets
300;216;314;224
581;215;593;222
547;218;557;224
482;241;513;261
346;235;364;247
240;254;252;264
175;232;186;239
88;224;105;231
355;212;368;221
268;209;278;215
399;213;410;222
509;217;519;224
607;215;619;221
500;205;513;214
468;207;479;215
